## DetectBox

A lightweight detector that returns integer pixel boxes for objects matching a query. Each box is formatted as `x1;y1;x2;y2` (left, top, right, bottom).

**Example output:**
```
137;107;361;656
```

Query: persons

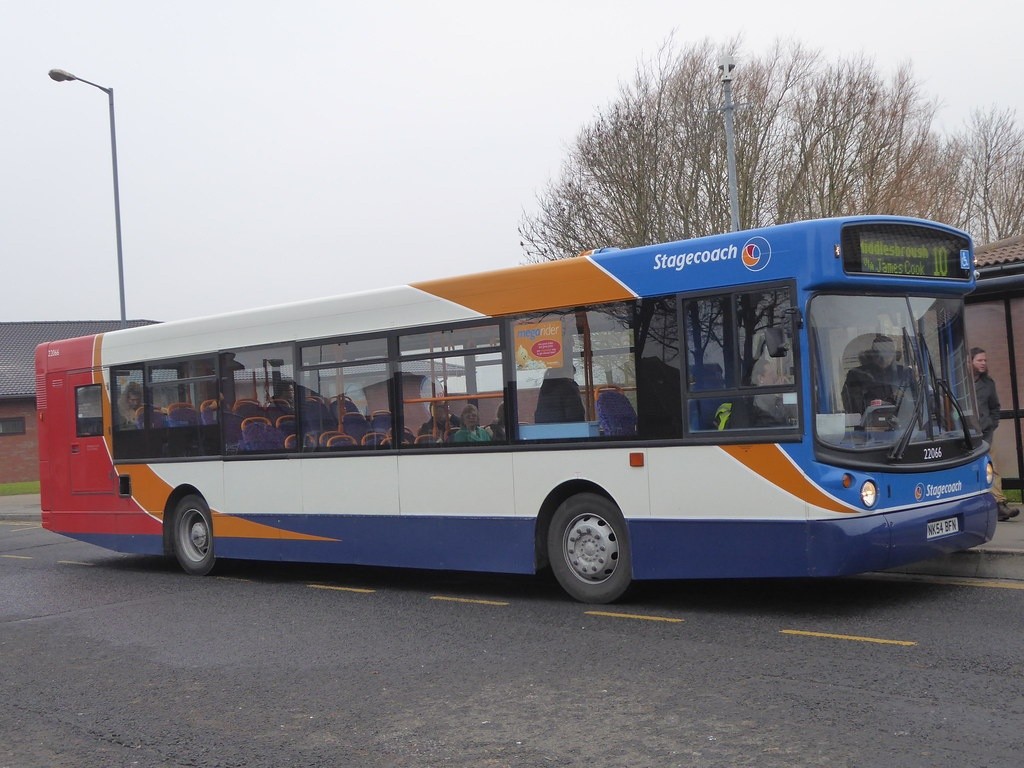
741;360;791;429
264;380;299;433
839;335;920;419
968;347;1019;522
417;401;509;445
534;363;586;424
116;382;143;430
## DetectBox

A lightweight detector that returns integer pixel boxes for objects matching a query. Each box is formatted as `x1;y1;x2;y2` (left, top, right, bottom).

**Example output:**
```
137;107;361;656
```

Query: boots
997;502;1019;522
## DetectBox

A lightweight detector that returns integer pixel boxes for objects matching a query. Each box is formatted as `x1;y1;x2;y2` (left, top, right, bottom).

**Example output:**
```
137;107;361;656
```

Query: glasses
466;414;476;419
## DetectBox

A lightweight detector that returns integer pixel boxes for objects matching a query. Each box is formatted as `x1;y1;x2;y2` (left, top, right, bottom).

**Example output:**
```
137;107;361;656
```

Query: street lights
719;52;741;235
49;69;126;329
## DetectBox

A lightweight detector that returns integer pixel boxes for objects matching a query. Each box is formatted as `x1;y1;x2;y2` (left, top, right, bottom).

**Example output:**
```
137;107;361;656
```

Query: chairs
136;393;460;450
593;385;638;438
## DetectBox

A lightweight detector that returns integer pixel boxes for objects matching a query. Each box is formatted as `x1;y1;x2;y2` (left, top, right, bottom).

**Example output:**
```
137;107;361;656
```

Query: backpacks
711;399;733;430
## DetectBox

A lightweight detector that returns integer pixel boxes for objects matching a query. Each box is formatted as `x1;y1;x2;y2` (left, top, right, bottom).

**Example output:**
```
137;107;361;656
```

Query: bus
34;217;1000;603
